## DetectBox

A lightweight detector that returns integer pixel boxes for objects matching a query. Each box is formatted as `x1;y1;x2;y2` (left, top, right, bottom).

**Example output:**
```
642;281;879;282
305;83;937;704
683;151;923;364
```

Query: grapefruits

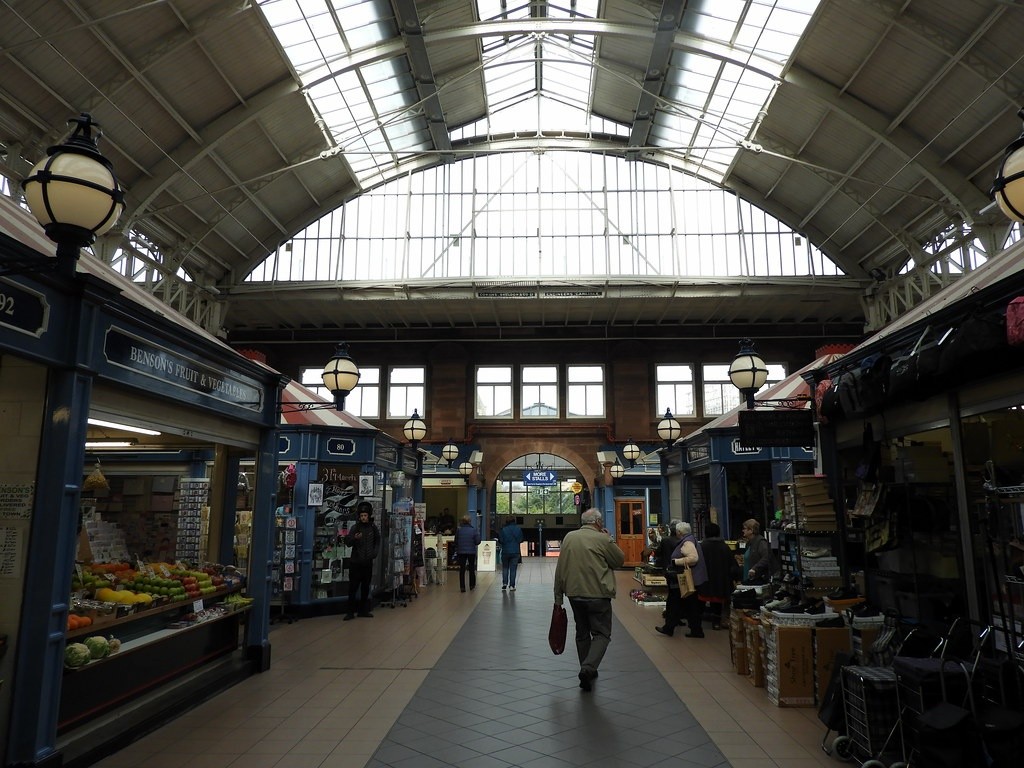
97;587;152;604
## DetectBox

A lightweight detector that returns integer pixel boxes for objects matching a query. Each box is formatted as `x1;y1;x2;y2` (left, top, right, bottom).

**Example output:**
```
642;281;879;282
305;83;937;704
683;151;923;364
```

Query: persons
342;502;380;620
739;519;778;585
654;519;686;626
655;522;709;638
439;508;455;531
700;523;740;630
553;507;624;690
453;515;482;593
498;515;523;590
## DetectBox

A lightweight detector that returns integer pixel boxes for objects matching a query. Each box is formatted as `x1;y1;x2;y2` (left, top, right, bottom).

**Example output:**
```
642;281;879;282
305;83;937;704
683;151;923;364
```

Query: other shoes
655;626;674;636
343;613;354;620
712;623;720;629
470;586;475;589
509;586;516;590
460;588;466;593
592;669;598;678
685;631;705;638
729;541;880;628
578;669;592;691
357;612;373;617
502;587;507;590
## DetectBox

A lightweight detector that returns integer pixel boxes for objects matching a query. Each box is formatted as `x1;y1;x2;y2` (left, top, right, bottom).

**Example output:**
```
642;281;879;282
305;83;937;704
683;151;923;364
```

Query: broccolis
65;635;111;664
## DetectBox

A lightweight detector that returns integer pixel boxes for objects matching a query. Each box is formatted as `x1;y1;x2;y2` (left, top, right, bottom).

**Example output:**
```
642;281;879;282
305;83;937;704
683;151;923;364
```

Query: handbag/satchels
548;604;568;654
83;463;110;491
518;552;522;563
850;464;951;559
677;559;696;598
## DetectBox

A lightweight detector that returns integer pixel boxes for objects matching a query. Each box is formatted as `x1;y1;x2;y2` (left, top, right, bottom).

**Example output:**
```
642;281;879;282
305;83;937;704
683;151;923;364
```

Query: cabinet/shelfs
762;482;848;605
866;457;1024;711
630;575;666;607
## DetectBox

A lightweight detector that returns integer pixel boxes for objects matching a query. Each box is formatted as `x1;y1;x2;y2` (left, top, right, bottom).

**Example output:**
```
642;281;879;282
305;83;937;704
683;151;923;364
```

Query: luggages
816;605;997;768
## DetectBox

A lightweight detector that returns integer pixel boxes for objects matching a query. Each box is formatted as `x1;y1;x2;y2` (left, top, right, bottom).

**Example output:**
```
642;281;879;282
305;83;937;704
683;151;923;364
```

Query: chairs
891;611;996;768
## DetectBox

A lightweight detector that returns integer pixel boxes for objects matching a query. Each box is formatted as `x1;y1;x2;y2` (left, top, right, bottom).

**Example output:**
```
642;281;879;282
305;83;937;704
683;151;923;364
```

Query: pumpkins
67;613;91;631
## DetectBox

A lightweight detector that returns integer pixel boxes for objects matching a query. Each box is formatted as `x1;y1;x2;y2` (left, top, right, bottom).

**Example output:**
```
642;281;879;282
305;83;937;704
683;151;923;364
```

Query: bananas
226;592;255;606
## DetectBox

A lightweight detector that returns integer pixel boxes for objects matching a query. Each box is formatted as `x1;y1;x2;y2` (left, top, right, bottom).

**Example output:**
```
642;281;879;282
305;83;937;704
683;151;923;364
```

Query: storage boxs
767;625;815;707
814;626;850;705
791;475;836;531
853;628;881;651
728;610;765;687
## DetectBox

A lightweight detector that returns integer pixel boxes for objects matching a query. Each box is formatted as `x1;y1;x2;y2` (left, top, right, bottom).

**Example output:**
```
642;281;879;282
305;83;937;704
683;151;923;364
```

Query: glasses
743;527;749;530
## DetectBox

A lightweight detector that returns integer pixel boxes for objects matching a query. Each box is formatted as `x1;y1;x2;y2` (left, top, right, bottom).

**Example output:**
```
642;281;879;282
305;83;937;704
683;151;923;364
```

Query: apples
73;562;139;591
127;567;227;602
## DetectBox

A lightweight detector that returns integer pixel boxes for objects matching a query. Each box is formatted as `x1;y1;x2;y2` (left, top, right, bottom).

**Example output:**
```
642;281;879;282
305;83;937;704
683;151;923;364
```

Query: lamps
988;105;1024;226
278;340;360;413
375;408;427;455
423;437;460;469
622;435;660;468
657;408;710;453
609;457;625;480
459;456;473;484
0;111;127;283
84;437;139;447
728;337;812;412
